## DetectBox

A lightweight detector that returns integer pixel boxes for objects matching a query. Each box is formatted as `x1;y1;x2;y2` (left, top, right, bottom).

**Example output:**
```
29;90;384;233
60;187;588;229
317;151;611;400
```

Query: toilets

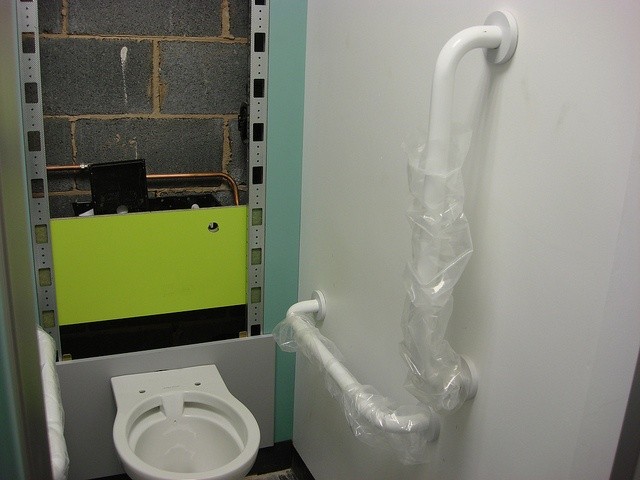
110;364;261;479
